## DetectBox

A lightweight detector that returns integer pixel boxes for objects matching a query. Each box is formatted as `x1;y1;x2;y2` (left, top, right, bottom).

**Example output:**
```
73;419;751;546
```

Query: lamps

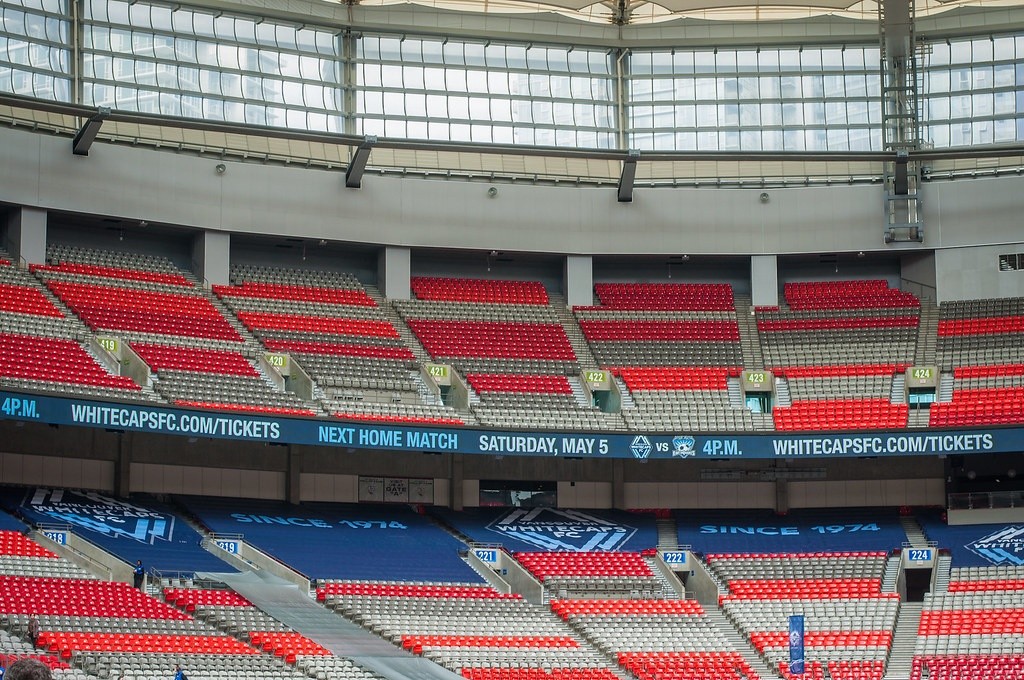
138;220;148;229
760;193;770;203
319;240;328;248
487;256;491;273
488;187;498;196
489;251;499;257
682;255;689;262
858;252;866;259
302;247;306;261
120;224;123;242
216;165;227;174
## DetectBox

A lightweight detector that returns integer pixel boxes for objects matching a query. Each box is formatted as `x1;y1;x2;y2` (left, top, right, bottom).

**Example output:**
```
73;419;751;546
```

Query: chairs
0;527;1024;680
0;241;1024;432
